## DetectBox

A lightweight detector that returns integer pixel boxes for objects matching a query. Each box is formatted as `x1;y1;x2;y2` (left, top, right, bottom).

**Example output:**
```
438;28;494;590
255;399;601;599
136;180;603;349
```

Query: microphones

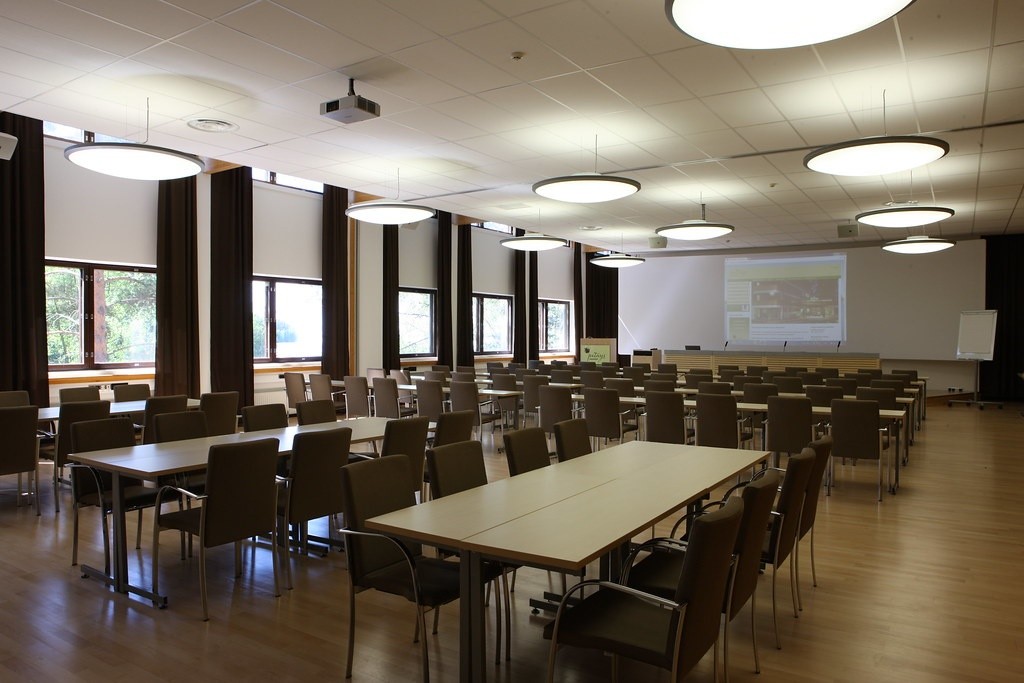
783;340;787;352
837;341;841;352
724;341;729;351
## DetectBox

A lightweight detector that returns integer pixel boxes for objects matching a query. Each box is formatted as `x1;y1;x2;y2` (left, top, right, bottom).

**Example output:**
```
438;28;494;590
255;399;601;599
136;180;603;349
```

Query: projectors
320;95;380;124
649;236;667;248
838;224;859;238
0;132;18;161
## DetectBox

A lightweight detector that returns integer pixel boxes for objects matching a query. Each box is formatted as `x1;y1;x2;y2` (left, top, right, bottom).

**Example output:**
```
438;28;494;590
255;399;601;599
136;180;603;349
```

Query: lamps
880;225;957;254
655;205;735;240
802;91;950;177
63;98;204;180
533;134;640;202
346;166;435;225
500;210;567;251
589;233;645;267
856;172;955;228
667;0;915;49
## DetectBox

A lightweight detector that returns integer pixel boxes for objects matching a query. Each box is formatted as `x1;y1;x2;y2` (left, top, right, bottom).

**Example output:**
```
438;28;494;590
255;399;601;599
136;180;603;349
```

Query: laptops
685;345;700;350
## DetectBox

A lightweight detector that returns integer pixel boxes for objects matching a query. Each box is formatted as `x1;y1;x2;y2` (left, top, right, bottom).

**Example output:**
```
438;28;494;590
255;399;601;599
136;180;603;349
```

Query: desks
39;350;931;682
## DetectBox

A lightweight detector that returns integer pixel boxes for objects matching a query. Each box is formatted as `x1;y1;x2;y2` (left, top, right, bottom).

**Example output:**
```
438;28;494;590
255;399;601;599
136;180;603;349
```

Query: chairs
0;361;920;683
